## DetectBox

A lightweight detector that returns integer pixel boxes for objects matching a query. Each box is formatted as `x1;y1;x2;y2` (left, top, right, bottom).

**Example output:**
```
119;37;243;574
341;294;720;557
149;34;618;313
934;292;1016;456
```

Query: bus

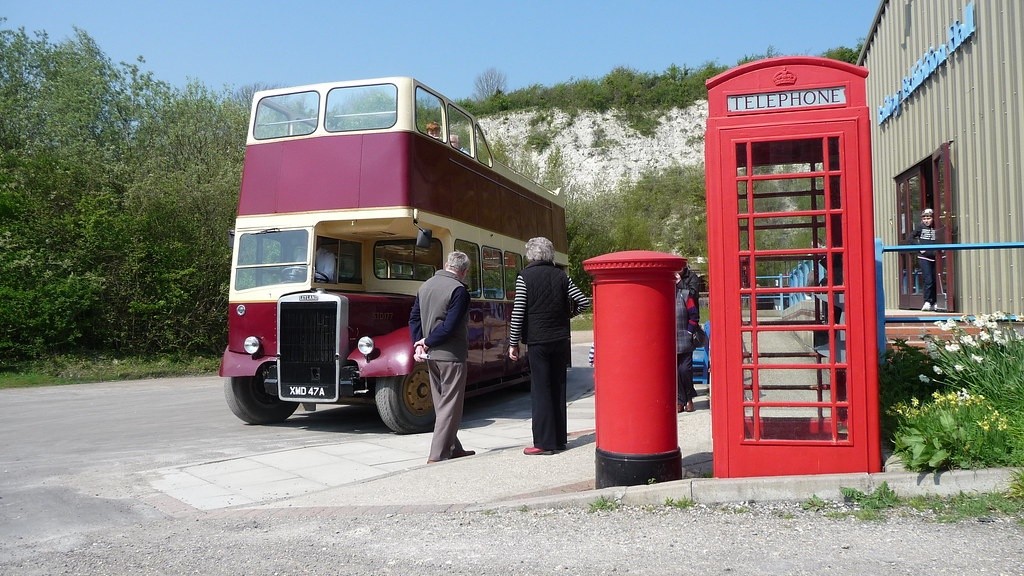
218;76;570;434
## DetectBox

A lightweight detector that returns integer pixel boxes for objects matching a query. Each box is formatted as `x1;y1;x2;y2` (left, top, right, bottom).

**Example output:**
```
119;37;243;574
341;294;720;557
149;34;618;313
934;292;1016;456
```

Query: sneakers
933;304;941;312
921;301;933;311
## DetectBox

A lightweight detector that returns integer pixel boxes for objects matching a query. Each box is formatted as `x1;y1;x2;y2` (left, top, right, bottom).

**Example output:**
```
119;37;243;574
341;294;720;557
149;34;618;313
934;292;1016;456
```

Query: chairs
693;321;710;384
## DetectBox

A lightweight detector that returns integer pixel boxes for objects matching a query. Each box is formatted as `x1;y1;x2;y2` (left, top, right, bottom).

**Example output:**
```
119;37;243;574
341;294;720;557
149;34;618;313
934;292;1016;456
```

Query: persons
906;208;937;311
291;237;337;281
409;252;476;464
675;268;700;413
450;135;470;156
509;237;590;455
426;121;440;139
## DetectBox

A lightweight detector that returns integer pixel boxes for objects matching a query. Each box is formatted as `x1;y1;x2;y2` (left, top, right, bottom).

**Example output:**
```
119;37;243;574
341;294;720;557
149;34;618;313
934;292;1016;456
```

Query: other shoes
524;444;566;455
426;460;441;464
448;451;475;460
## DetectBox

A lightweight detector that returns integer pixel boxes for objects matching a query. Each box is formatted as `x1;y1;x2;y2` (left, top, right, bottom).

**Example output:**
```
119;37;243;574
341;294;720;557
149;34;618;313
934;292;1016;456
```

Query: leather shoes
678;404;685;412
686;399;694;412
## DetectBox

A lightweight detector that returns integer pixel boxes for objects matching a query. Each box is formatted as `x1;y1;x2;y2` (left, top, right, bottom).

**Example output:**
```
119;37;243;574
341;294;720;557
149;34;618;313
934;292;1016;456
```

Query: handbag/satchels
692;326;708;348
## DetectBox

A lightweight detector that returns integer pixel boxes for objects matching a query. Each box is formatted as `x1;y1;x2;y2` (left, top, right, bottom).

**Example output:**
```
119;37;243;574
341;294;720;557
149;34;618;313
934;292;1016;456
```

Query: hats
921;208;934;217
